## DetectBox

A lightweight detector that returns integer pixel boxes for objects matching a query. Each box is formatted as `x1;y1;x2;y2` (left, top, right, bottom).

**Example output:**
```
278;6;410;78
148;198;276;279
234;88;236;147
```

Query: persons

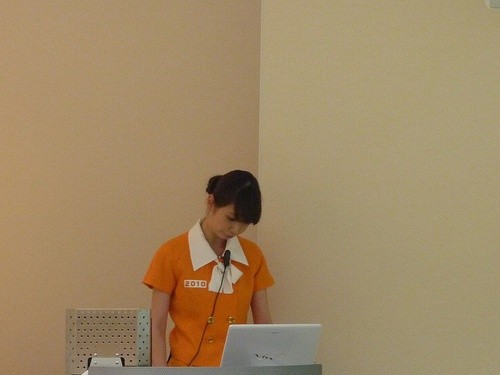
141;169;275;368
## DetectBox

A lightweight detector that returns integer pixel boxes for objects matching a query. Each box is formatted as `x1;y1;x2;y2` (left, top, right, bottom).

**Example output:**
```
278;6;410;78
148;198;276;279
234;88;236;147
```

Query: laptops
219;324;322;366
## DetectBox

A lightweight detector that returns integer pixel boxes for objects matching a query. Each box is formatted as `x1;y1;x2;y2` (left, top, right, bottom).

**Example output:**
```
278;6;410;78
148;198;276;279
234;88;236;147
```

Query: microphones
224;250;231;271
